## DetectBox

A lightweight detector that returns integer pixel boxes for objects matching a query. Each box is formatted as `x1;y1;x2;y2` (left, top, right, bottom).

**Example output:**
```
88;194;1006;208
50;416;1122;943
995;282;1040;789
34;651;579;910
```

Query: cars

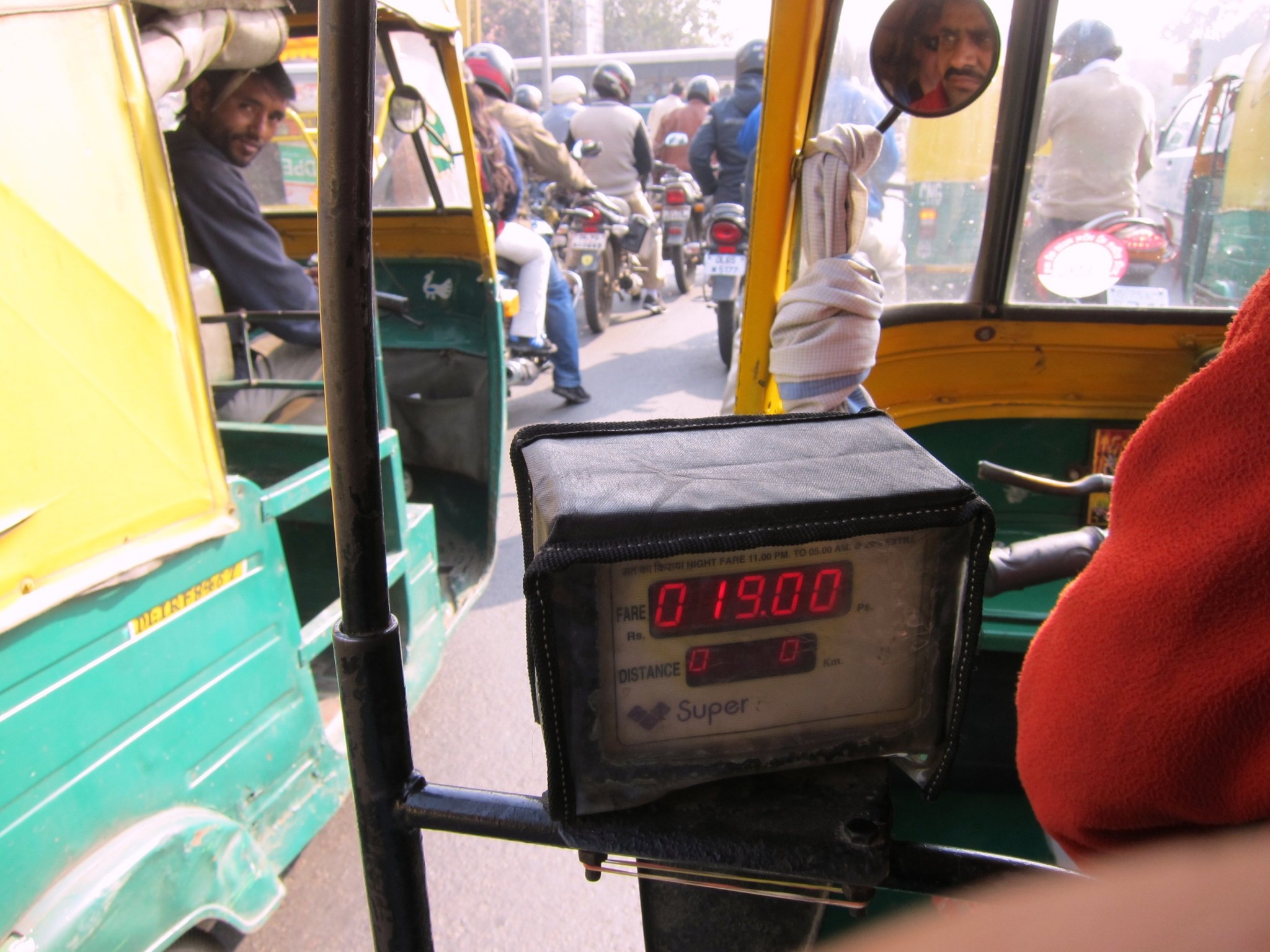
1126;72;1245;290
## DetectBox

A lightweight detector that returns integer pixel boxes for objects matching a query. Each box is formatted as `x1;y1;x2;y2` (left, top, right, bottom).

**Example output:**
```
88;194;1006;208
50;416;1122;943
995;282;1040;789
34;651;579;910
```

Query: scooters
1012;206;1171;309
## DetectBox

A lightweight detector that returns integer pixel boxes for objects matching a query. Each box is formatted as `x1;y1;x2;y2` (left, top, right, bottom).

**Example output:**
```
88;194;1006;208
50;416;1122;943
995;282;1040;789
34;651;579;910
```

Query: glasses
913;31;939;52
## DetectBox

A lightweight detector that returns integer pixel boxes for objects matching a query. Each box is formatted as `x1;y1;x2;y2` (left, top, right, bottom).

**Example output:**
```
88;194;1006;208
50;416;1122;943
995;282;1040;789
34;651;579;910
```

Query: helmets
512;84;543;111
592;59;636;101
462;42;518;103
686;74;721;105
733;38;767;77
550;74;587;106
1047;17;1123;82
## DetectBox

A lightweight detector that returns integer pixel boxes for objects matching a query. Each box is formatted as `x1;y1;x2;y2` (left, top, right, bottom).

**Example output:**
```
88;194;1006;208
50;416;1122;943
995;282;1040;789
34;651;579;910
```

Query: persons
799;255;1270;951
1017;16;1158;287
874;0;994;114
162;59;412;504
369;36;899;403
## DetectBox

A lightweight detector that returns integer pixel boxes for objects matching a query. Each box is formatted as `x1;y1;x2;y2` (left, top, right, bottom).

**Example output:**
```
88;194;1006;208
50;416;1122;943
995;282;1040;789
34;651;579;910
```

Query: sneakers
642;293;663;314
551;384;591;403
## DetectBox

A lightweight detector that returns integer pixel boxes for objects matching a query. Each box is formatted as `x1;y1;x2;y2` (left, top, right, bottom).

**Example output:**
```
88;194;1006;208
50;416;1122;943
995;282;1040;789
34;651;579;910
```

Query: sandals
506;334;559;355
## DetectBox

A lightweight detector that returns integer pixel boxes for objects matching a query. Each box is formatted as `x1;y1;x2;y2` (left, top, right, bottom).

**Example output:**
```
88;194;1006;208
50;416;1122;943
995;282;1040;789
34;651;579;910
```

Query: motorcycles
702;202;754;369
487;133;710;335
3;1;518;952
